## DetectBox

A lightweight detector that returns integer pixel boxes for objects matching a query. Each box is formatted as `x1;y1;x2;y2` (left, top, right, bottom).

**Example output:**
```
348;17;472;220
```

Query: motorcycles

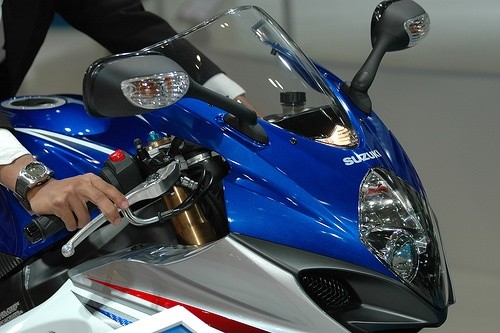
0;0;457;333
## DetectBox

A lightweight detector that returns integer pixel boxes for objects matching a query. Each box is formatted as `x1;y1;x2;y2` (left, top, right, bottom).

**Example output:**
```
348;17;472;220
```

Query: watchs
13;162;55;208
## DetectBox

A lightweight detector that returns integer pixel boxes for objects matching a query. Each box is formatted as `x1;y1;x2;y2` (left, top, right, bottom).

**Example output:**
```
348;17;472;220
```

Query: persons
0;0;264;231
